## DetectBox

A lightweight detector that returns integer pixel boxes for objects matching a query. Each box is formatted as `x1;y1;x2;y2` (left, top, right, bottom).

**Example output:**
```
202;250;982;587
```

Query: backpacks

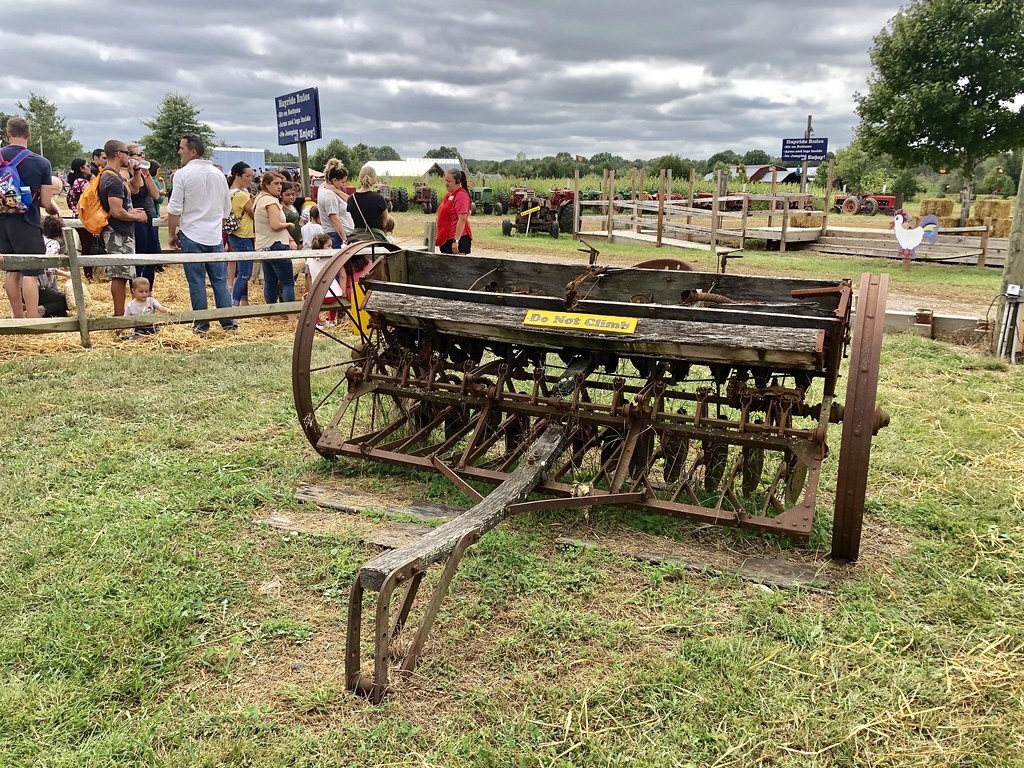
76;166;125;237
222;190;246;235
0;149;38;214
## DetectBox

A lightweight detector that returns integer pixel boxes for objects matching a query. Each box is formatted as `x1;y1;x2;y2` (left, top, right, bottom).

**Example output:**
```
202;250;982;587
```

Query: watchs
453;239;459;243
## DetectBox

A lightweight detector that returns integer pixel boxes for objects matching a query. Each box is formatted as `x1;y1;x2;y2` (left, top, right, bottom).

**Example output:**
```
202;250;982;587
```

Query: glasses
115;150;128;156
130;151;143;156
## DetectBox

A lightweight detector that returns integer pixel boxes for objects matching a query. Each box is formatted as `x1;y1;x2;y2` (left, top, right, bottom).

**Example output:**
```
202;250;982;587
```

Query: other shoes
315;323;323;332
324;321;335;328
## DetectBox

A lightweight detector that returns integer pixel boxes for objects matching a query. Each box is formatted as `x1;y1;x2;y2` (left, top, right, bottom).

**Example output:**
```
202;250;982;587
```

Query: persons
24;131;394;335
434;168;472;255
0;117;52;320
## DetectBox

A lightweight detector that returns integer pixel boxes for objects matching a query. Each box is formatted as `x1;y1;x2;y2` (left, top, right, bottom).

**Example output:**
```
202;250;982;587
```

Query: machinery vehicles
373;181;399;212
828;191;898;217
497;176;536;215
468;175;502;216
399;178;438;215
551;187;753;236
501;193;559;239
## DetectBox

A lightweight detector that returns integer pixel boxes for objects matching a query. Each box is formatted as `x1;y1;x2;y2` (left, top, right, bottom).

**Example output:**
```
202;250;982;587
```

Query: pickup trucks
309;178;356;204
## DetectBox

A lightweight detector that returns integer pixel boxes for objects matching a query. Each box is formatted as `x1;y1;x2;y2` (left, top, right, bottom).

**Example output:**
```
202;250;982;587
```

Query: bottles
128;158;150;169
20;185;32;204
